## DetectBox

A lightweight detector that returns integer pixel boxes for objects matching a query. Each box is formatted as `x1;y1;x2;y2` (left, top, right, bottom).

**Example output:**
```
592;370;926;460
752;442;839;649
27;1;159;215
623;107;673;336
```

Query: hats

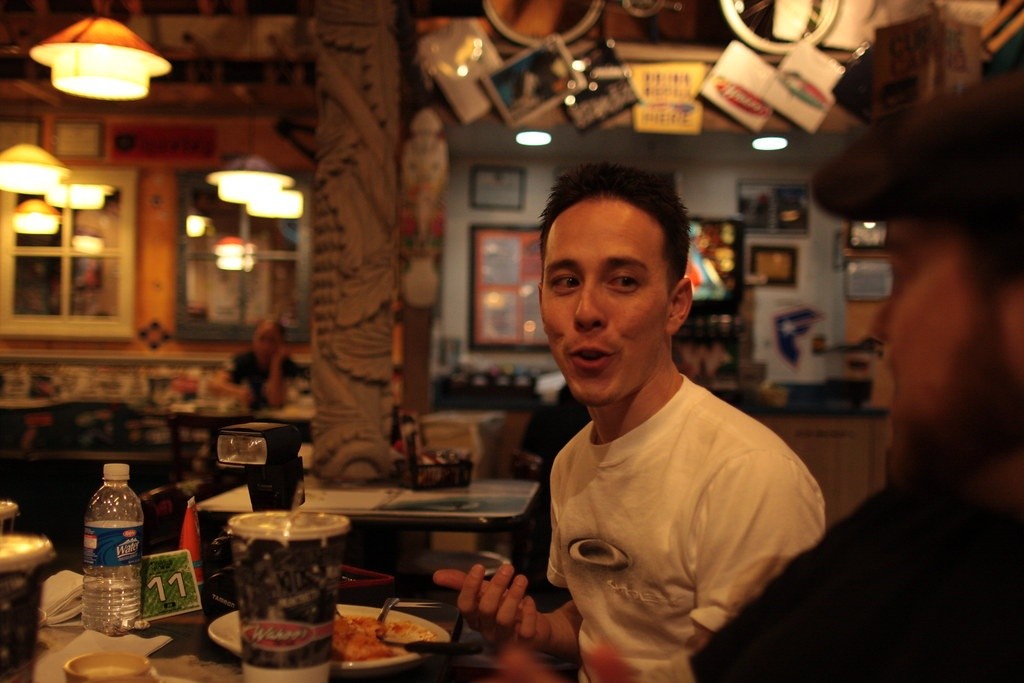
810;70;1024;277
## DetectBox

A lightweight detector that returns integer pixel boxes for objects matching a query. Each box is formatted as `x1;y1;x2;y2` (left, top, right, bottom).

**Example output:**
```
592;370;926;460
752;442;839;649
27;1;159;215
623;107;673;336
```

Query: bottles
80;463;144;636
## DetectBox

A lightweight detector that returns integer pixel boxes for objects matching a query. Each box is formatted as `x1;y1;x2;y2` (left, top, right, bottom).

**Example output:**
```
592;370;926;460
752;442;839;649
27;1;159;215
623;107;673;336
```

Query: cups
228;511;350;682
0;534;55;682
63;651;152;683
0;500;18;535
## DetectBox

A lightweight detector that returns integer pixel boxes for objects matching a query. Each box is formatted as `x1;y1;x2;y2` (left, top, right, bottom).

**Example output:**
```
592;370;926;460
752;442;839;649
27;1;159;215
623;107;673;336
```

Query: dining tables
0;394;544;683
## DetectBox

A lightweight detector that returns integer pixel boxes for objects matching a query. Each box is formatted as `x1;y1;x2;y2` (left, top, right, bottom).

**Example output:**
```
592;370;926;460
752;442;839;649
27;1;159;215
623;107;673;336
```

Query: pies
334;615;397;662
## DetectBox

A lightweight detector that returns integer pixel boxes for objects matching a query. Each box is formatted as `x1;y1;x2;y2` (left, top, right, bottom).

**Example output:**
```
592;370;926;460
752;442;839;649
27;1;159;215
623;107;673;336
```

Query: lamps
0;101;73;194
215;421;302;468
212;234;256;256
10;199;63;234
29;11;172;102
43;166;114;210
206;147;295;205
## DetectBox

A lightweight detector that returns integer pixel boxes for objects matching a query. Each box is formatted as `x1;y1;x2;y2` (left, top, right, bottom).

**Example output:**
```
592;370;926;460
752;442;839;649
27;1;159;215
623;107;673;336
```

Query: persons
432;164;825;683
230;320;299;409
686;72;1024;683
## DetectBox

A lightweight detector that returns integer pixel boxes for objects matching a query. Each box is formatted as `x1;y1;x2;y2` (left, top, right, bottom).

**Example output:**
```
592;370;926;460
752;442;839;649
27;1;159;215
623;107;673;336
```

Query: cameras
197;421;340;623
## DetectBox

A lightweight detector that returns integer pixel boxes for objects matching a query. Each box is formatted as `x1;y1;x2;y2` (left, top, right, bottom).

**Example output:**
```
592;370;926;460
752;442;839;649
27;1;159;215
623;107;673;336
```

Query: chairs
165;409;258;477
384;406;514;644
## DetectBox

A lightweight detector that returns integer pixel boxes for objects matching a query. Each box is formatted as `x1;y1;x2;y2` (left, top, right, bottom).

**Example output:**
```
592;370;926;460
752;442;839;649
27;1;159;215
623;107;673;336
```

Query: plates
208;603;451;675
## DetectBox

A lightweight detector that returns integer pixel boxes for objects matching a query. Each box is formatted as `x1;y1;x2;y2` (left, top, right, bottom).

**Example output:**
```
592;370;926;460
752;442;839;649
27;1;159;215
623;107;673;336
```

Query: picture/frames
467;164;526;211
0;115;43;149
467;223;552;353
750;242;797;288
53;119;106;160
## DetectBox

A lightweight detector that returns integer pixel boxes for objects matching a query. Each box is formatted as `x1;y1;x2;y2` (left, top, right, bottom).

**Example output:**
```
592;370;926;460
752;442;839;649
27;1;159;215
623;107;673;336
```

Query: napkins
56;630;173;664
